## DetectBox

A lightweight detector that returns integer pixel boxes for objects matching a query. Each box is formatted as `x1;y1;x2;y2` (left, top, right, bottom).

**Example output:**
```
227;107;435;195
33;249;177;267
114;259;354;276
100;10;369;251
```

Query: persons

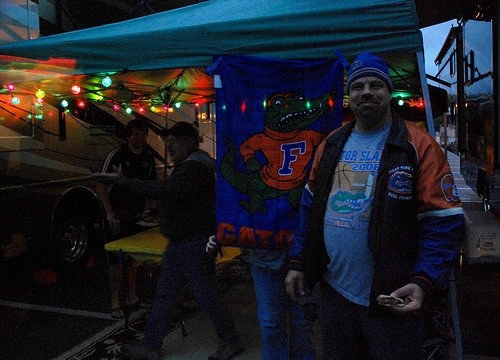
284;52;466;360
129;121;245;359
97;118;156;318
205;236;317;359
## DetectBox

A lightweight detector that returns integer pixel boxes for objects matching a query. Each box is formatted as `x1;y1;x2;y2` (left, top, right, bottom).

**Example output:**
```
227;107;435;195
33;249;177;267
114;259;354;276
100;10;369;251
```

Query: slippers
110;305;125;318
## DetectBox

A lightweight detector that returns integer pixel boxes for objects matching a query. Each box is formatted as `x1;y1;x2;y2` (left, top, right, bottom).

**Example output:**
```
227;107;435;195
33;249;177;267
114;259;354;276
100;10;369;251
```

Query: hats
161;121;198;138
346;54;394;93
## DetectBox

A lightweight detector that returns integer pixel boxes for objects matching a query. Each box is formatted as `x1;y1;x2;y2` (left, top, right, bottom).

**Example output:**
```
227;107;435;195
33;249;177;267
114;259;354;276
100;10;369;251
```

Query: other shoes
124;347;161;360
207;341;244;360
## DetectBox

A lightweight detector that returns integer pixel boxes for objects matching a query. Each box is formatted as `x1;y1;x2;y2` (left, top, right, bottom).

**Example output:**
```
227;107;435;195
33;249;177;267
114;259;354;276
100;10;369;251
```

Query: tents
0;0;464;359
